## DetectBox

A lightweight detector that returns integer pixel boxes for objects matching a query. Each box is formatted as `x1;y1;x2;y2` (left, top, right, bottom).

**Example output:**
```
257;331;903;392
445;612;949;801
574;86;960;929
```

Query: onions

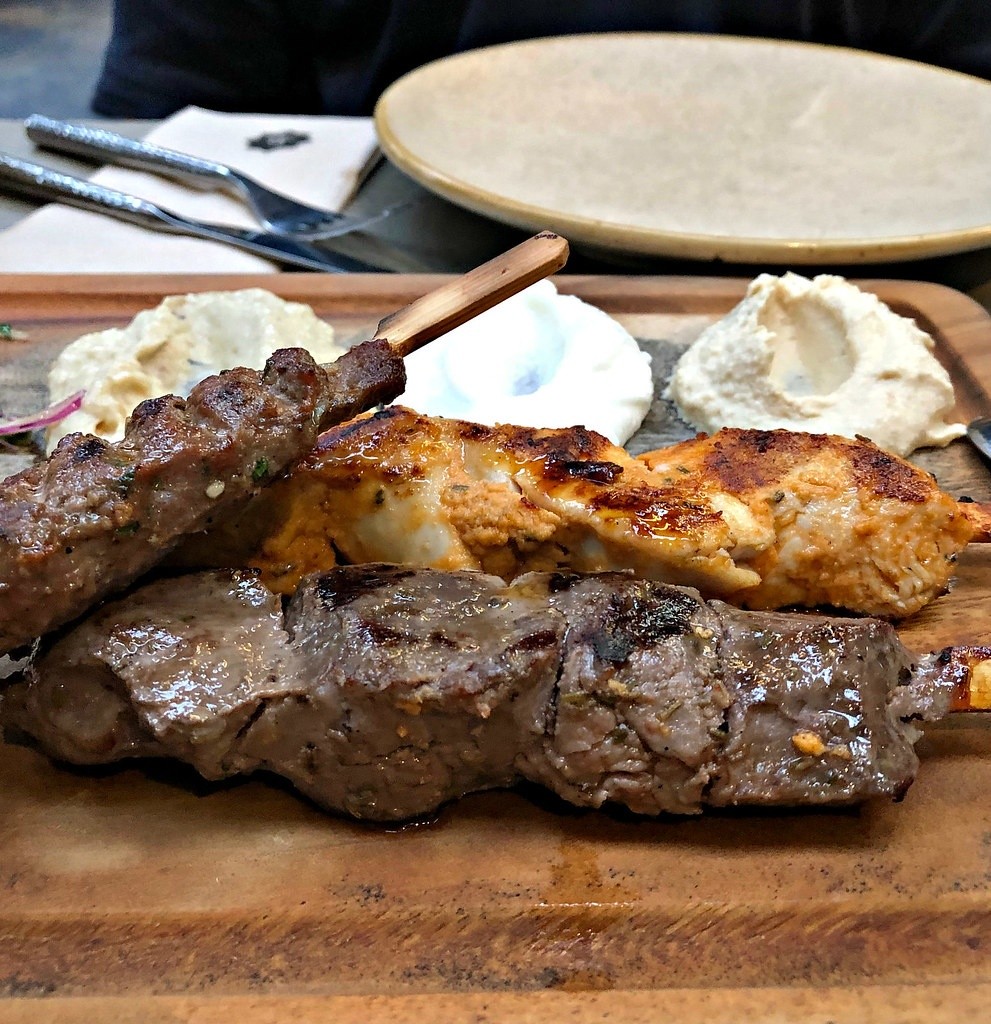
0;389;85;436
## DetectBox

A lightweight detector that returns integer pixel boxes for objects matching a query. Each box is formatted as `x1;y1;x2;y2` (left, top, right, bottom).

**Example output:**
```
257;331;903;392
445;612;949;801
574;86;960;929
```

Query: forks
19;108;413;243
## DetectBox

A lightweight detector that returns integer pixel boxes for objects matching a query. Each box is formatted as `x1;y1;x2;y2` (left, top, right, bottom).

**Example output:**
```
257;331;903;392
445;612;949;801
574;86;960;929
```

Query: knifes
0;152;402;276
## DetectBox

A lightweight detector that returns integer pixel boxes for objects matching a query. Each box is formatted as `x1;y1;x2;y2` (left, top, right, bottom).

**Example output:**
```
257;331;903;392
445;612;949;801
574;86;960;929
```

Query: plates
374;31;991;266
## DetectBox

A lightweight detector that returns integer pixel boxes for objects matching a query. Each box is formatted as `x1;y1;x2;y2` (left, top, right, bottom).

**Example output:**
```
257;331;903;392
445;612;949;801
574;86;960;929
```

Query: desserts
661;271;970;466
39;287;351;453
388;276;652;452
